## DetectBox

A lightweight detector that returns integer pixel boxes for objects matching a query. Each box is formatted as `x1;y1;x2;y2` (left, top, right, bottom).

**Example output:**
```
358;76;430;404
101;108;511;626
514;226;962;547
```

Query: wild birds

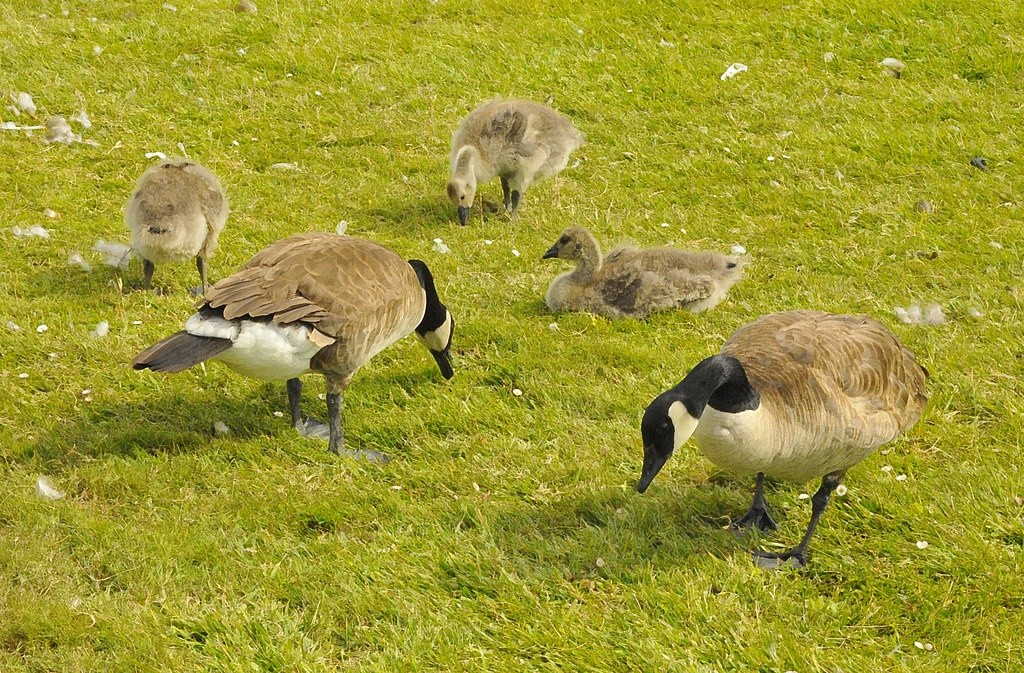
444;95;591;228
635;312;931;576
539;223;755;319
121;153;232;298
129;234;454;466
